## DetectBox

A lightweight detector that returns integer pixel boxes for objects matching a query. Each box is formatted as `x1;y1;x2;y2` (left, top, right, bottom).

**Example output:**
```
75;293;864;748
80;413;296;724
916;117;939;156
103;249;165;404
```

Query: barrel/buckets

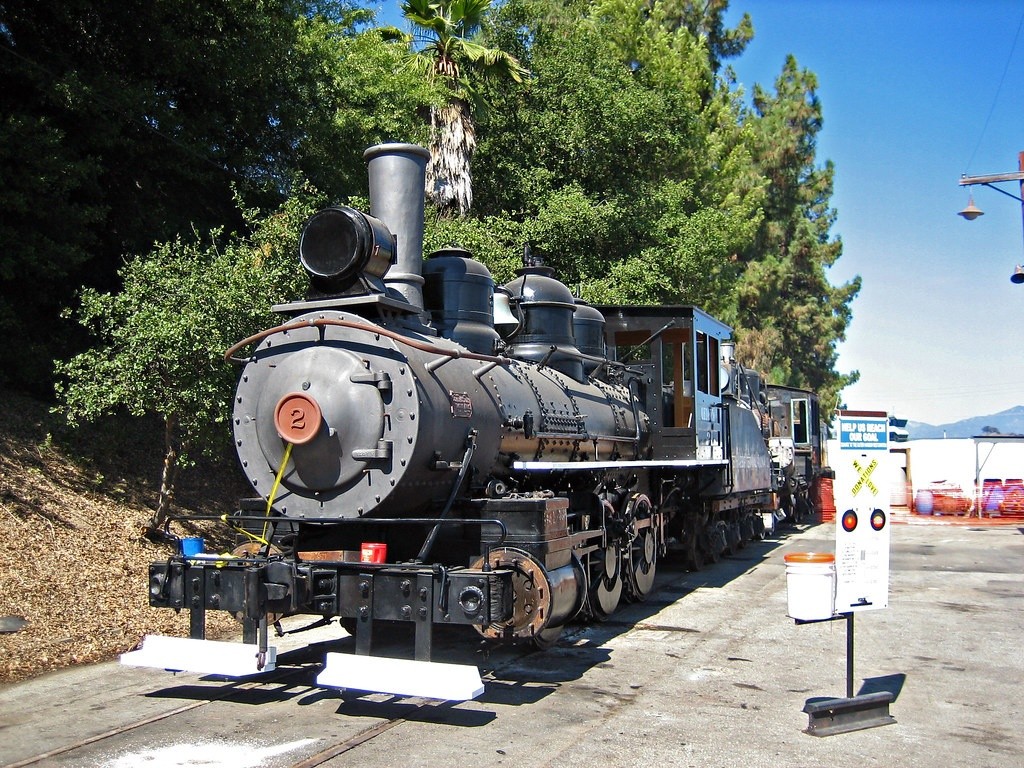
1003;479;1024;516
982;479;1004;517
915;489;933;515
814;478;836;523
183;539;204;557
361;543;386;567
784;553;835;620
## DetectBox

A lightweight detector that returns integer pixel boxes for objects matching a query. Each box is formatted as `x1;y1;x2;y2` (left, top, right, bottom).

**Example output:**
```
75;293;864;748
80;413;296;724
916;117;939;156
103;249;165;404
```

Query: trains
118;144;821;702
